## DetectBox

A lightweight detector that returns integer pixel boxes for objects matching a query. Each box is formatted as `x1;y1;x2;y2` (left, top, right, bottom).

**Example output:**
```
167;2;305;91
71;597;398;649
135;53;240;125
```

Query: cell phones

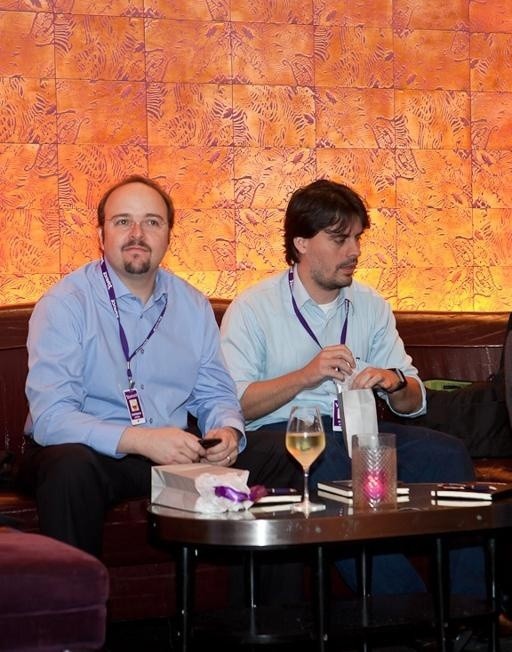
199;438;221;448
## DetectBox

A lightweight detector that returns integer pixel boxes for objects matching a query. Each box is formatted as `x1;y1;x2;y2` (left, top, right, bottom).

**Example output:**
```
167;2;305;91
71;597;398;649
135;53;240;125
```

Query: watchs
377;368;408;394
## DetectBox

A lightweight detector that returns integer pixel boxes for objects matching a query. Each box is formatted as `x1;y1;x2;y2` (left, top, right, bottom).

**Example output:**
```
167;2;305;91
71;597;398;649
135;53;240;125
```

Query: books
317;489;410;505
430;481;498;499
250;486;303;503
431;498;493;507
249;504;293;513
317;480;410;498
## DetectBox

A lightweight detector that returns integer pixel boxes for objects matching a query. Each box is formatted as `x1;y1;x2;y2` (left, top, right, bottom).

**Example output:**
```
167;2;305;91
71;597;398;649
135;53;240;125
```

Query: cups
352;433;397;514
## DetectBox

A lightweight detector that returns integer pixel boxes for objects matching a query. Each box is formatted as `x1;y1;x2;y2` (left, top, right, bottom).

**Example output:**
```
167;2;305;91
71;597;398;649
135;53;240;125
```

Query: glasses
103;218;169;229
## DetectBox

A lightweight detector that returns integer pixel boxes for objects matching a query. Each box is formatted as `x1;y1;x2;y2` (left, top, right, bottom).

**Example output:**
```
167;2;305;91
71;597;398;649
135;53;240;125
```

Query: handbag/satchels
446;380;512;461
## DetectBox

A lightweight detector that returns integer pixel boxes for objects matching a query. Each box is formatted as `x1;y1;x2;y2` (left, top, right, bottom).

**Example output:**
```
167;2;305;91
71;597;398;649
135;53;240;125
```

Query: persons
26;174;248;562
222;179;489;597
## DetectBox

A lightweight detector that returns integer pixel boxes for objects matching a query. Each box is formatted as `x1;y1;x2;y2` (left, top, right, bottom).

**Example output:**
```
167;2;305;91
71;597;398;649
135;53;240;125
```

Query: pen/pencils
265;488;298;494
436;482;498;491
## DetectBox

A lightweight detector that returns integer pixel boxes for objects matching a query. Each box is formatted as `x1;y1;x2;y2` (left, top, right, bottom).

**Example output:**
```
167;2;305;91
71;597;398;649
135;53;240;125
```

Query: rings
226;455;232;464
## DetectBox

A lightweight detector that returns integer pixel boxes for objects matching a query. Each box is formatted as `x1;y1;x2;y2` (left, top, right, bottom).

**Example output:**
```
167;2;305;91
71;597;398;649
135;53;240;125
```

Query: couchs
0;299;512;651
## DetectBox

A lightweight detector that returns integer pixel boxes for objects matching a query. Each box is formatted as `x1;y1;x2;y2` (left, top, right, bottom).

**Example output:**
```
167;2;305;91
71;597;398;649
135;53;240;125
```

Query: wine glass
285;407;326;513
